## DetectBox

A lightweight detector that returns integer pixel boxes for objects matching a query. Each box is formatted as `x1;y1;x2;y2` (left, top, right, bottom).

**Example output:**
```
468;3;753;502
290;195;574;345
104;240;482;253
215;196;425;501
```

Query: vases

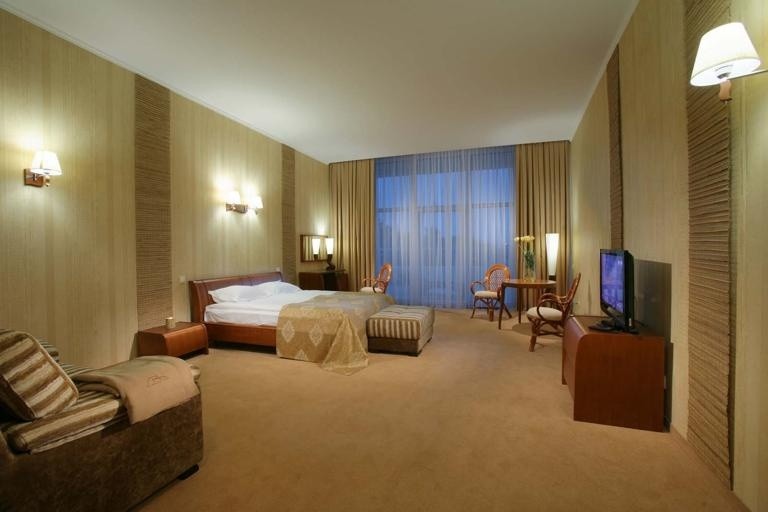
523;252;535;281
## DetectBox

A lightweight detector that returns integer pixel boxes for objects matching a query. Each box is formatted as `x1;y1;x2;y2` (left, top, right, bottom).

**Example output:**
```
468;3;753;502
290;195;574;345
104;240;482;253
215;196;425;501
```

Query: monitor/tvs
599;249;635;332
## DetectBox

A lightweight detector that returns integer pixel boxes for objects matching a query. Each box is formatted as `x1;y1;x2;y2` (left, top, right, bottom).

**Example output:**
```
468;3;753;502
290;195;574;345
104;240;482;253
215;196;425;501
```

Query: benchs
366;304;435;357
138;320;209;356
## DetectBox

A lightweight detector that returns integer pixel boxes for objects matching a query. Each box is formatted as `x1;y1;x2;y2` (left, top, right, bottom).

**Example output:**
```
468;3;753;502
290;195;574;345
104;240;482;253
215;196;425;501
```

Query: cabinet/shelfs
562;316;665;433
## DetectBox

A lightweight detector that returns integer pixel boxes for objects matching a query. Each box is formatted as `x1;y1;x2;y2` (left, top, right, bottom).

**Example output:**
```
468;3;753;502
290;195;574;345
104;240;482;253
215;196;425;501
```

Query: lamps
23;149;62;187
325;237;335;271
225;191;264;215
545;232;559;280
690;21;768;105
311;238;320;260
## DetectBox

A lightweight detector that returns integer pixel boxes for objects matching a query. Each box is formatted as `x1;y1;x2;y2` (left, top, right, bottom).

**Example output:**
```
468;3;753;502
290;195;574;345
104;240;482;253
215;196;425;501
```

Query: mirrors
300;233;328;262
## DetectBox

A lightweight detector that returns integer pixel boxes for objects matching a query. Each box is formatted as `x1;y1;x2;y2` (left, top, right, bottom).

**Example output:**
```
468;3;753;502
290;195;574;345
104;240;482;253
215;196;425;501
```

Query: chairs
360;263;391;294
470;264;512;322
0;337;204;510
525;272;581;352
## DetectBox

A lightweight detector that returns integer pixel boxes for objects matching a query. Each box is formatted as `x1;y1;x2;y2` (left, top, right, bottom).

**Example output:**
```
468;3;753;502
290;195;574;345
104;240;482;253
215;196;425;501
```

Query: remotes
589;325;611;331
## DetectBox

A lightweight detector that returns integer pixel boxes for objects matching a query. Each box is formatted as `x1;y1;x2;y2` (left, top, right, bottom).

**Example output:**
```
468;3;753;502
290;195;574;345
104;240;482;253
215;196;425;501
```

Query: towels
71;356;200;426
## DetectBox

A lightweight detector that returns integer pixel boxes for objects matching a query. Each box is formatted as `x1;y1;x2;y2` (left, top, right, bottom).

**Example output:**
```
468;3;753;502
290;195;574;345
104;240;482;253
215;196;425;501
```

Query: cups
165;317;175;328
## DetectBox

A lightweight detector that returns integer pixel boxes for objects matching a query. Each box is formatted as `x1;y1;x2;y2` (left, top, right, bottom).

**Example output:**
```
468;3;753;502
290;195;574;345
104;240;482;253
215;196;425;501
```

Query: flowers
514;235;535;278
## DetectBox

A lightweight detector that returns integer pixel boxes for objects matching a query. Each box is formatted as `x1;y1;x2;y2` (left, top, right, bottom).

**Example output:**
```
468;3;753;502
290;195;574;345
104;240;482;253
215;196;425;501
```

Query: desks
498;277;557;329
299;269;349;290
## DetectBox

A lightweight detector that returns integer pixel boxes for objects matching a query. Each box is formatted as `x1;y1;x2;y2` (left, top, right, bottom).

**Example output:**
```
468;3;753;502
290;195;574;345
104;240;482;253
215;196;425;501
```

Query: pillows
208;285;268;305
252;280;301;296
0;327;81;421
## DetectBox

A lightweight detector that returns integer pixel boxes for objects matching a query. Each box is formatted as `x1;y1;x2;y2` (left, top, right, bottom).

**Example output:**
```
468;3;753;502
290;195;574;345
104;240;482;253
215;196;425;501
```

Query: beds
189;272;396;376
